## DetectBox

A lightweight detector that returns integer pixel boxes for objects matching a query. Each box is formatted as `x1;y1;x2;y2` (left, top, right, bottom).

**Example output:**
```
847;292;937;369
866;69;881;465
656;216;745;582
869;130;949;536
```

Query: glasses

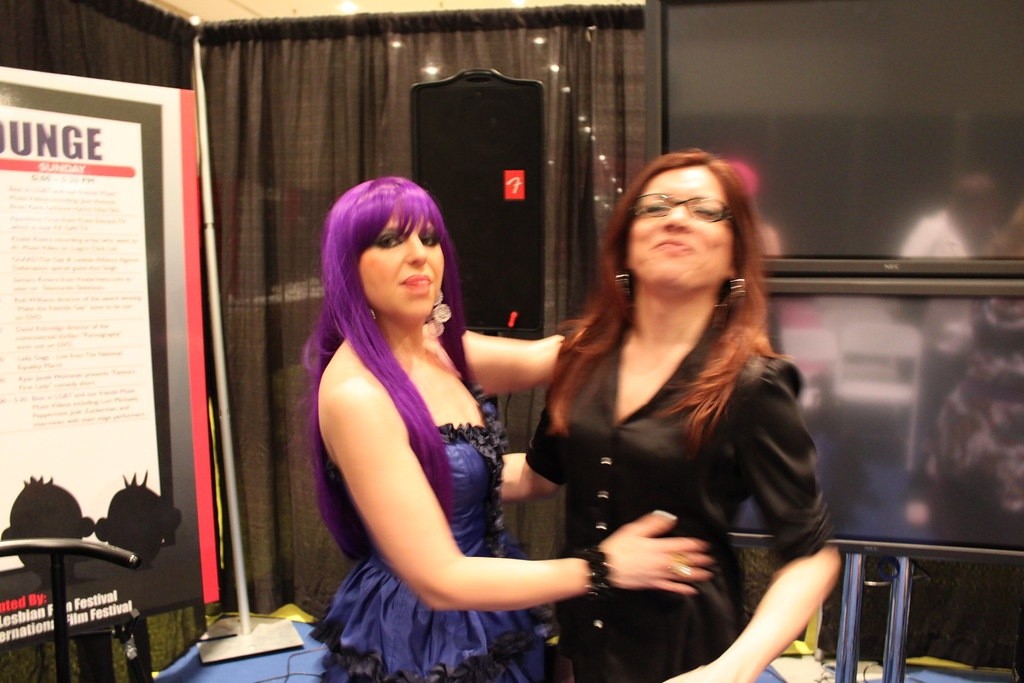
631;194;734;223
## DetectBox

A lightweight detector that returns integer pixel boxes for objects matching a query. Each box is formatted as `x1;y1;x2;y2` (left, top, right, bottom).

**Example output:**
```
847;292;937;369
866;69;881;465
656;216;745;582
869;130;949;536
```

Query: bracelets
577;545;615;592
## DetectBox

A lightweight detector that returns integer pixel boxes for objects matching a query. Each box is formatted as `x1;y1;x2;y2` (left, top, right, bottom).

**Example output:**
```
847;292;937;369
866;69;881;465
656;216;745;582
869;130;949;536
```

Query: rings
675;565;691;577
676;553;687;565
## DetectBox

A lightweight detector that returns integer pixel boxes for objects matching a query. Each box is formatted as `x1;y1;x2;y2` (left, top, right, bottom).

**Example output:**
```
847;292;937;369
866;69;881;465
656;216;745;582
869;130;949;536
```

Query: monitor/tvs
723;279;1024;565
648;0;1024;279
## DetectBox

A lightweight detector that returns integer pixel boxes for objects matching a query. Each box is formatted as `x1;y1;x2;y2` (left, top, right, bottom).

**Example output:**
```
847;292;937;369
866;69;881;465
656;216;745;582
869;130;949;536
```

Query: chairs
828;324;923;470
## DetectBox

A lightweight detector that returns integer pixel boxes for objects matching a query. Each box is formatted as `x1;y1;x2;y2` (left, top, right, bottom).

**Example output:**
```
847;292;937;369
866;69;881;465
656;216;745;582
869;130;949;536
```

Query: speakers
409;68;547;334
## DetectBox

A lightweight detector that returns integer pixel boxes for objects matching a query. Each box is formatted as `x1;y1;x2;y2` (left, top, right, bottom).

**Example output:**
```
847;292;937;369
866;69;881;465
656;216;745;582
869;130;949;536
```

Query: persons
301;176;715;683
795;367;835;435
500;148;841;683
